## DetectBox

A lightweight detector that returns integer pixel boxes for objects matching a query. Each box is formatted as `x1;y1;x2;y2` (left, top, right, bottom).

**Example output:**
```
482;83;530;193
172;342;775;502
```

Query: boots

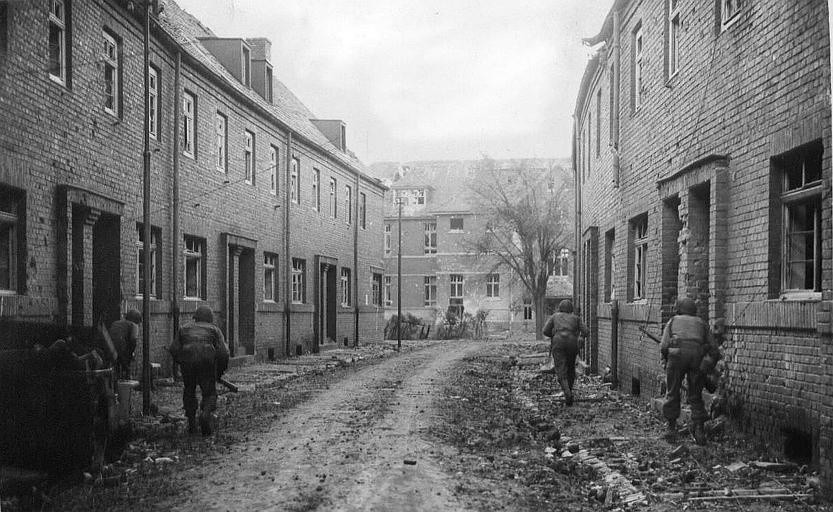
189;414;197;433
560;378;574;406
664;418;677;444
200;409;211;435
694;422;707;446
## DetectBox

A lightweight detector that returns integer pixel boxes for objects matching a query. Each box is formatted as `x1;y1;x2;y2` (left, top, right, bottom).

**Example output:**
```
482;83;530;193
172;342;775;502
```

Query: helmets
677;298;696;314
193;306;214;323
558;300;573;312
127;309;143;324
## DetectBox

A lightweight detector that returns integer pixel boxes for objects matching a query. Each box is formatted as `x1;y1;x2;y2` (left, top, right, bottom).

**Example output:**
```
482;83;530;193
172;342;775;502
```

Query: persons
169;304;230;435
543;298;590;405
109;309;141;375
659;297;721;446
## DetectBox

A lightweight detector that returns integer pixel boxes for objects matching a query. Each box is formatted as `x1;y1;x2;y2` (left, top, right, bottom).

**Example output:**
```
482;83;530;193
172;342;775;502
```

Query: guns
638;327;718;394
164;345;238;393
